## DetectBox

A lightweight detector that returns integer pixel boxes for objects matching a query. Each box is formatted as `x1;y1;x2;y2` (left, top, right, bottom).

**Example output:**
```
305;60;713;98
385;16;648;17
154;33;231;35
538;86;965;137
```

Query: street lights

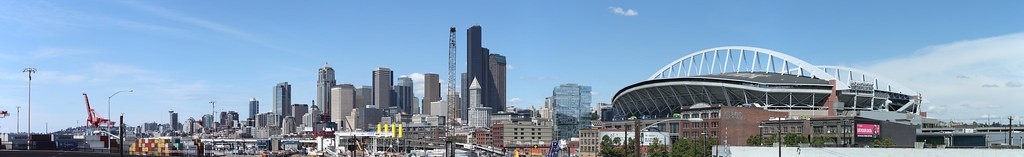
16;105;22;135
209;101;216;119
107;89;133;155
22;67;37;149
